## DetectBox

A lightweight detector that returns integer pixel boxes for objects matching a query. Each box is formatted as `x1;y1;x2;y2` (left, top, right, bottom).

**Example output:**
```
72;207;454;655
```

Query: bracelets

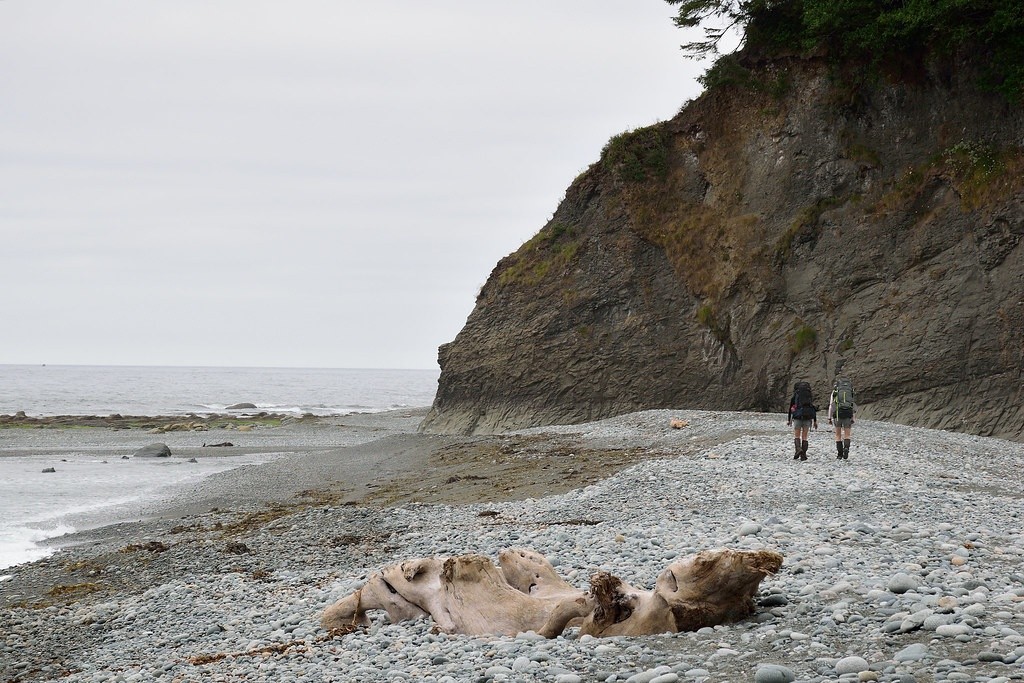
828;418;832;420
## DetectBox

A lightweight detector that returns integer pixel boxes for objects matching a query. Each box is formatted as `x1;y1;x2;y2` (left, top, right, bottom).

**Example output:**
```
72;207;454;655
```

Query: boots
844;439;850;459
794;438;809;461
836;441;843;460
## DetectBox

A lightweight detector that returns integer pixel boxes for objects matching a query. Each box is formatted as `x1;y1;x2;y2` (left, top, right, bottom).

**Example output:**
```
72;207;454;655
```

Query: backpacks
791;382;814;420
832;378;853;418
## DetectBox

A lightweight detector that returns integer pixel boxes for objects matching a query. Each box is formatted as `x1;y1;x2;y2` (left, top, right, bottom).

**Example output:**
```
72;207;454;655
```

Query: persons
787;392;818;460
827;382;857;459
